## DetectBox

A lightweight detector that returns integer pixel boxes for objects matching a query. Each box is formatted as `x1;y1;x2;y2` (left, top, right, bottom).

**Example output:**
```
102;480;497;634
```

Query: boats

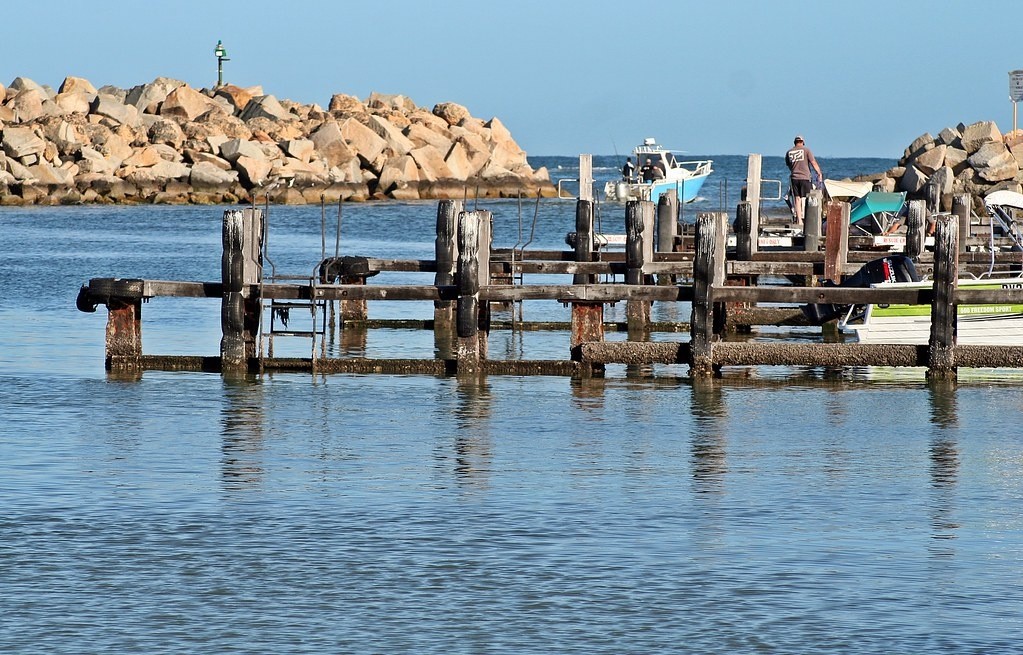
604;138;714;201
837;190;1023;345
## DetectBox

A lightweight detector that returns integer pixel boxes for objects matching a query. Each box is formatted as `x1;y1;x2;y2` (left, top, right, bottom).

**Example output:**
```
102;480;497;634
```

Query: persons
883;198;936;237
641;159;657;184
785;136;823;226
623;157;634;183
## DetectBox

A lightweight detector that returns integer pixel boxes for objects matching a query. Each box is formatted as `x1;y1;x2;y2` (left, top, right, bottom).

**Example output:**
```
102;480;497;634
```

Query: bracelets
928;232;932;234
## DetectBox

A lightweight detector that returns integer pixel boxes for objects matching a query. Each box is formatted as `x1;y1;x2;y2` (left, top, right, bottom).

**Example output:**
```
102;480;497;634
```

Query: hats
795;136;805;146
